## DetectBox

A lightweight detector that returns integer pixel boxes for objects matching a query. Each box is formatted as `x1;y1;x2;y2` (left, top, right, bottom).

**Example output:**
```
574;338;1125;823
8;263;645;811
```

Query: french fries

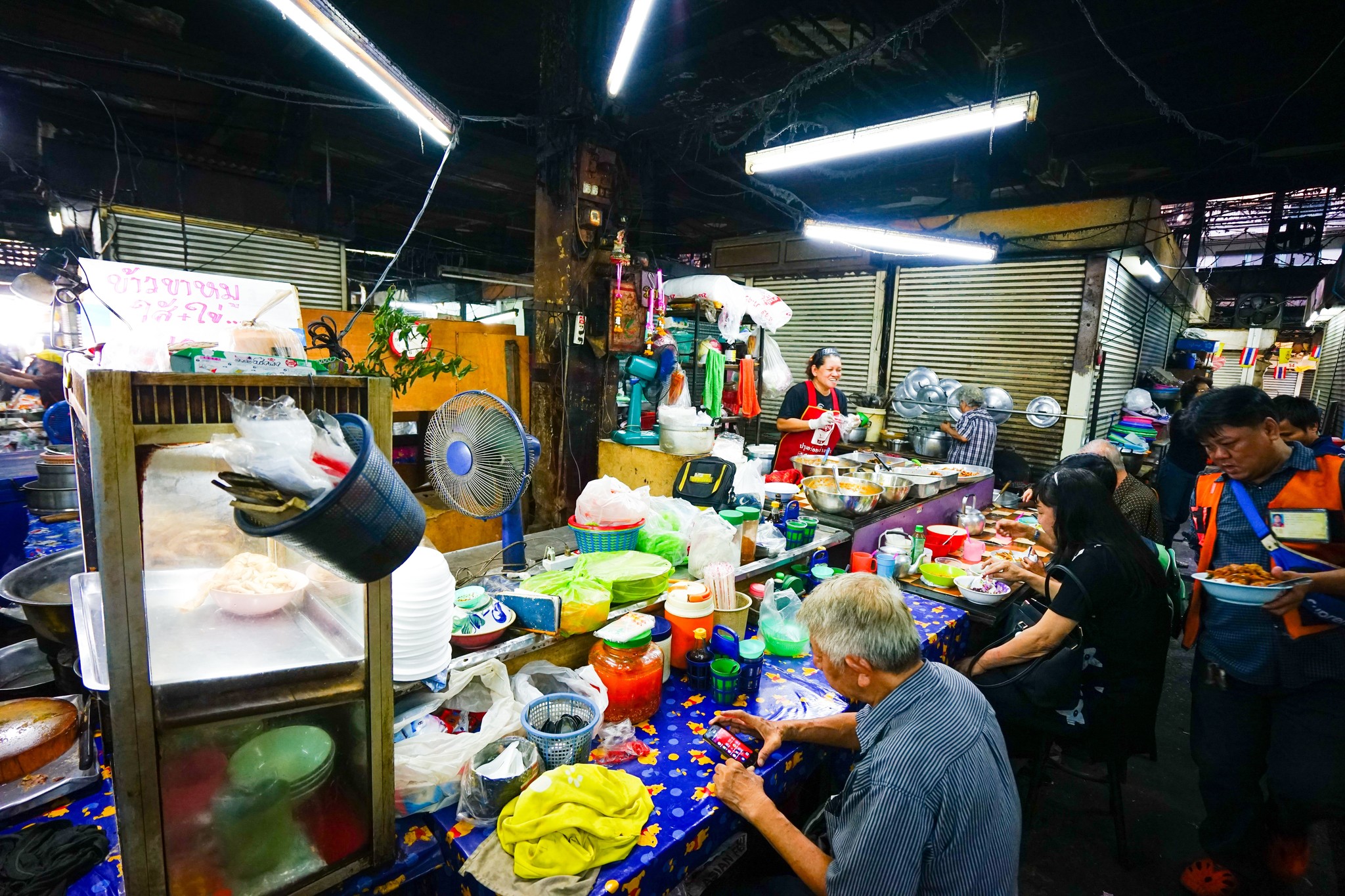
996;550;1023;562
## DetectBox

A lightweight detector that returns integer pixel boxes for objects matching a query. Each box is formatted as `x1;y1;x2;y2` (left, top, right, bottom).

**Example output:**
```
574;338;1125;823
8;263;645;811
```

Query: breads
0;697;80;787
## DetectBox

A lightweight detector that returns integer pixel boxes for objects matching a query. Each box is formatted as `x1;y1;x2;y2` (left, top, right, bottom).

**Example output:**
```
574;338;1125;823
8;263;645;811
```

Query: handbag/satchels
1261;534;1345;627
967;566;1089;705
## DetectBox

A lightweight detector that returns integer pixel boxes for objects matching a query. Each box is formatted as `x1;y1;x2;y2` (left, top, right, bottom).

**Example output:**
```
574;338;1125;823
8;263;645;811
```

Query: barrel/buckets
731;455;748;486
1181;353;1196;369
885;539;912;555
664;582;714;668
856;407;886;442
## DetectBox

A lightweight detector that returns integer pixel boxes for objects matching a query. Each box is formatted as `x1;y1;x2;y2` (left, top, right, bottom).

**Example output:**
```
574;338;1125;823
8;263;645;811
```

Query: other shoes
1270;838;1307;878
1181;859;1237;896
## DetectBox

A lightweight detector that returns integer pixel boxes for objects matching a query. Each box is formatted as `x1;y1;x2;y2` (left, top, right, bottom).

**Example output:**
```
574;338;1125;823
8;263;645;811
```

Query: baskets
232;412;428;585
568;515;646;554
521;691;599;768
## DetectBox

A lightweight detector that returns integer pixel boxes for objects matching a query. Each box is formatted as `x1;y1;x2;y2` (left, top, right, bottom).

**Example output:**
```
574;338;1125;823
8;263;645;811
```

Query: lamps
385;267;535;324
256;0;1042;267
9;246;70;307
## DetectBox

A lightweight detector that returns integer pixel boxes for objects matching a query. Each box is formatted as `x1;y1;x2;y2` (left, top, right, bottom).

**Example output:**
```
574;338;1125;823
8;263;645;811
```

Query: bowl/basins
811;566;834;579
590;550;672;599
204;569;309;616
160;725;336;814
1155;383;1179;390
880;431;908;451
391;547;517;682
919;525;1037;605
739;639;765;659
0;543;110;708
23;445;79;515
1191;572;1294;606
0;408;46;422
616;396;630;415
1105;407;1167;460
1018;517;1039;526
764;454;915;517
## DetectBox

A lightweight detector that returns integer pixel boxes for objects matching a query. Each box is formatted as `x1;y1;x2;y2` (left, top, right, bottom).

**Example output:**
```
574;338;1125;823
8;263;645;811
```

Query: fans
423;388;542;579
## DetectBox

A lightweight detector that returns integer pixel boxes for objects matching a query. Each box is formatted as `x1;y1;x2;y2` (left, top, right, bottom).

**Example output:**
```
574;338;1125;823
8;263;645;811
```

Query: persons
940;386;996;470
713;575;1024;896
957;378;1345;896
770;347;861;474
0;336;68;413
1272;513;1283;527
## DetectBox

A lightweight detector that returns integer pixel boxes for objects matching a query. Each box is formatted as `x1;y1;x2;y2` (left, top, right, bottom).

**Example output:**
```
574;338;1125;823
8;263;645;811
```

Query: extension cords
542;551;581;571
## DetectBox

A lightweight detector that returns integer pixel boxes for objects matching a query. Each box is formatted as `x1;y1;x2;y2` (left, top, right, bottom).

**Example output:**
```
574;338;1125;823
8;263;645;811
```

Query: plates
909;464;993;483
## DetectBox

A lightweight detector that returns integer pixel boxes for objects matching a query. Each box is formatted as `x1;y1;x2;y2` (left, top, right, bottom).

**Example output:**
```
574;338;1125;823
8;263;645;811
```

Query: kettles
950;493;986;535
875;531;927;578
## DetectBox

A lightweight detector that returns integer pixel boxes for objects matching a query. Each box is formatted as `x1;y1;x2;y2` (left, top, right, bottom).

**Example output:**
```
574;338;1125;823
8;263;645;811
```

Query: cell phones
703;722;755;767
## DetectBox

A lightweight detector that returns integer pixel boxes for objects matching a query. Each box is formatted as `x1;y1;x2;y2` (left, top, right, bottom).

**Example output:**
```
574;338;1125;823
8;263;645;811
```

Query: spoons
969;575;984;588
474;714;591;779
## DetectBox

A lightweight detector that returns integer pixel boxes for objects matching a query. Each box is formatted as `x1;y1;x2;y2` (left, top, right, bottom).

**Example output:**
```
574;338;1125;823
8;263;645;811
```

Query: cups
779;511;819;549
709;635;740;704
923;547;933;564
213;778;294;875
964;539;985;561
852;552;896;579
791;565;847;590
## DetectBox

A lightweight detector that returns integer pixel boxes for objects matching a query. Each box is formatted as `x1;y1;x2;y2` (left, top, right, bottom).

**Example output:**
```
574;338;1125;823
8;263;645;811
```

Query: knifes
79;695;95;771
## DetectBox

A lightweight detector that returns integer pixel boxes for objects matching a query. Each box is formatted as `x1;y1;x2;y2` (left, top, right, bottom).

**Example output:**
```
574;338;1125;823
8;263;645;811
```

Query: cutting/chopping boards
0;697;80;785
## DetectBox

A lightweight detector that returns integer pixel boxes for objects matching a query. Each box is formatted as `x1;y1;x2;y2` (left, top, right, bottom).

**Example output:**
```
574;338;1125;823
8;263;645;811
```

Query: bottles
719;506;760;566
911;525;926;574
816;546;826;559
765;494;783;524
587;629;664;725
724;343;737;365
690;628;712;687
774;572;784;592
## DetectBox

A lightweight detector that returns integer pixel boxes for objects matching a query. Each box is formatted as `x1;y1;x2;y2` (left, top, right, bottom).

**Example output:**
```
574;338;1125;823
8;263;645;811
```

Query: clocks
387;320;433;361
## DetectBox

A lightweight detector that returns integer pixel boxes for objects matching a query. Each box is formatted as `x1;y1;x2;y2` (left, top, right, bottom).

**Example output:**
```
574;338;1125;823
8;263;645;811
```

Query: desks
896;505;1056;649
1134;465;1153;490
1150;438;1172;474
429;588;971;896
0;724;444;896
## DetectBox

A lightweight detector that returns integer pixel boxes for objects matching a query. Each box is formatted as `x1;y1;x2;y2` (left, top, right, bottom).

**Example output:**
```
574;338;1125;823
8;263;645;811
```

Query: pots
656;411;722;455
743;442;778;476
1116;442;1148;475
849;421;872;443
992;445;1031;510
907;426;953;458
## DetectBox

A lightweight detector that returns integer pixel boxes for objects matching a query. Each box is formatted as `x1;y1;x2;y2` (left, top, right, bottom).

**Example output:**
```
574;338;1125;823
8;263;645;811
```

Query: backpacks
672;456;736;510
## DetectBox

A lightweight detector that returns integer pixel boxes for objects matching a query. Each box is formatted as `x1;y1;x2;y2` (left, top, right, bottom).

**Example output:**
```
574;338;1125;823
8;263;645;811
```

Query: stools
1019;730;1137;872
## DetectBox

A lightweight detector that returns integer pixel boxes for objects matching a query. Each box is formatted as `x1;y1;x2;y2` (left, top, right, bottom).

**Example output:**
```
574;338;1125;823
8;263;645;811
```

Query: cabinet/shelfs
64;348;396;896
666;293;765;447
